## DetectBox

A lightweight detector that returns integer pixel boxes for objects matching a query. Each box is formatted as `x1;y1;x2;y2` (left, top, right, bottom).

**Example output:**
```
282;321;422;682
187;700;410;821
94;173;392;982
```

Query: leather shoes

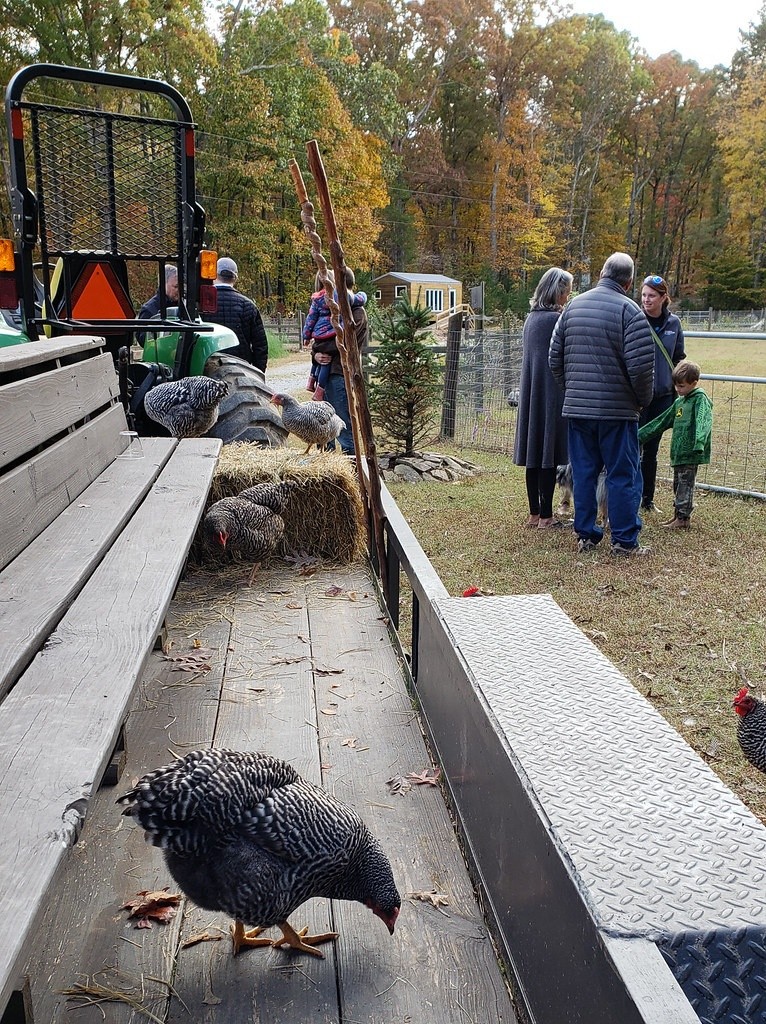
659;515;691;530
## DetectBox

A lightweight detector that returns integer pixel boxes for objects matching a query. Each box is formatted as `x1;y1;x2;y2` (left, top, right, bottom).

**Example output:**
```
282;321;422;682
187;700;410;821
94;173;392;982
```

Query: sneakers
610;543;651;558
577;538;603;554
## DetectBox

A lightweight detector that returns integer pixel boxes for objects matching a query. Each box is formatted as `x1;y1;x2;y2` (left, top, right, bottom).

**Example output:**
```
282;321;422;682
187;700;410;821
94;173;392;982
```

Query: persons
640;275;686;514
548;252;655;557
638;360;714;529
135;264;178;348
512;267;574;531
302;268;367;401
200;257;268;373
310;266;367;473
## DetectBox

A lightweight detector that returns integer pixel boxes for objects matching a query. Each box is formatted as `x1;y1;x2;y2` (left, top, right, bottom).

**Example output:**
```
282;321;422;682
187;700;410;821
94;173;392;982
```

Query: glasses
643;275;664;284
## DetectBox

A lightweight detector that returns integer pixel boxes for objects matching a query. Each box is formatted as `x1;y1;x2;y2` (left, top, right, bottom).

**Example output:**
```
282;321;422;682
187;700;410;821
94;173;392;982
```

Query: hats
345;266;356;286
217;257;238;278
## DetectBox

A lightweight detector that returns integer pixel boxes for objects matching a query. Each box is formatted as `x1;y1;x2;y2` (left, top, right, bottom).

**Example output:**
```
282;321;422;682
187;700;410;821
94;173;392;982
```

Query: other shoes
529;515;571;529
645;502;664;516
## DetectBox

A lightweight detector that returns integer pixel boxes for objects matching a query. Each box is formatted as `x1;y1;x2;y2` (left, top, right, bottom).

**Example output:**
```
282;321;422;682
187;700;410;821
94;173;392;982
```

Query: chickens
204;480;296;588
730;687;766;774
143;374;234;441
269;393;347;458
115;747;401;961
